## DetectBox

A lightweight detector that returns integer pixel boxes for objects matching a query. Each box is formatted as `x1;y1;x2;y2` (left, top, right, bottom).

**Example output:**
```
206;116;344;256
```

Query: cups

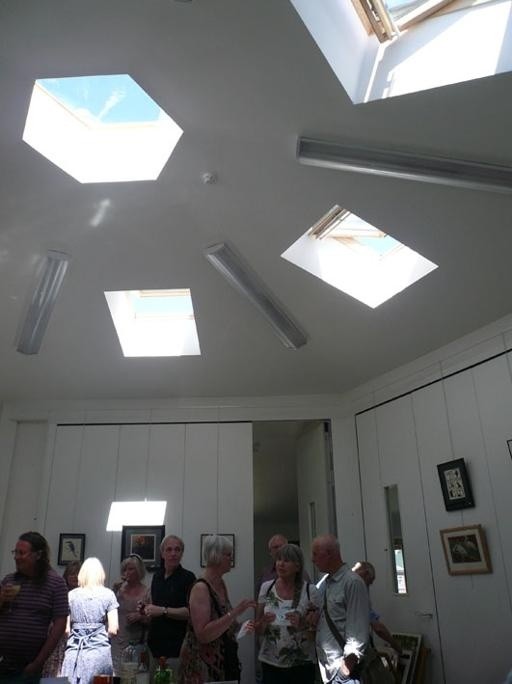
93;675;113;683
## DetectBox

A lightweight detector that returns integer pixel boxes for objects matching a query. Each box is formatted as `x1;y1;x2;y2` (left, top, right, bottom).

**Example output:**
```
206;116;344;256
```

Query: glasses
10;550;31;556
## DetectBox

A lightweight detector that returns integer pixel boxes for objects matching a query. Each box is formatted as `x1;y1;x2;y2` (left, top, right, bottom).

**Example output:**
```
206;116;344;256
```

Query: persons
0;531;403;684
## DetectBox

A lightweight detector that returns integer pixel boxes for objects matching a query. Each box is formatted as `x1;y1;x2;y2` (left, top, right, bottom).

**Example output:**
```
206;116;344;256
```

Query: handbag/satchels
220;635;245;684
351;647;401;684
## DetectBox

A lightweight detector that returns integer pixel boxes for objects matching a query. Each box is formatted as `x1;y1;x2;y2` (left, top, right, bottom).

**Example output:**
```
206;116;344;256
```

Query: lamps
294;136;512;197
203;238;309;353
17;248;71;356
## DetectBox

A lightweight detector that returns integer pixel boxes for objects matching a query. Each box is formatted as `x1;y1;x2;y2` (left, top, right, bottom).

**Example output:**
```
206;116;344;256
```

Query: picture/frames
438;524;494;576
119;525;166;573
200;532;235;569
57;532;87;565
434;456;478;513
386;632;432;684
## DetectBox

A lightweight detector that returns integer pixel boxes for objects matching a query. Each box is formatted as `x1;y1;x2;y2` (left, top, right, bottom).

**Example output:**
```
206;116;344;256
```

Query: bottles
110;638;177;683
